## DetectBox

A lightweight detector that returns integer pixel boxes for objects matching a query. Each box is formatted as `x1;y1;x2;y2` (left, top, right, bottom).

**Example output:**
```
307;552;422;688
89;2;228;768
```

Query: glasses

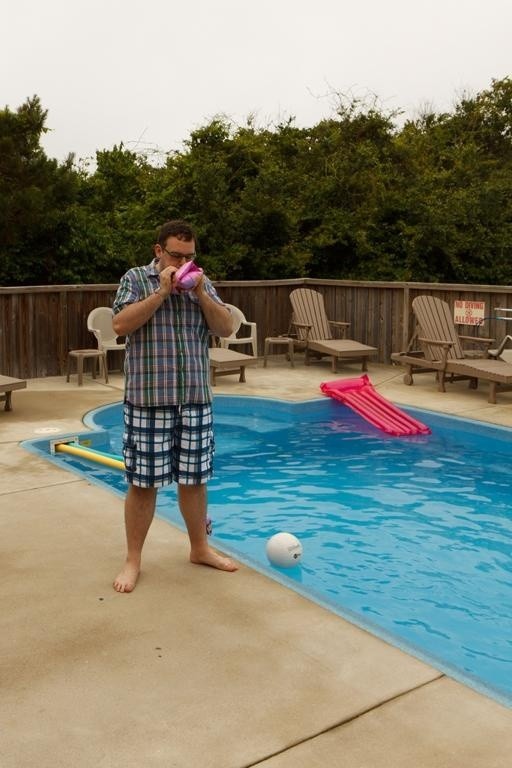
163;246;197;260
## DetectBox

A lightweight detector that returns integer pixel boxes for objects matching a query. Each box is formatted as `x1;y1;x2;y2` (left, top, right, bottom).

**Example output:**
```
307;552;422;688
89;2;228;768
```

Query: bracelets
154;286;168;301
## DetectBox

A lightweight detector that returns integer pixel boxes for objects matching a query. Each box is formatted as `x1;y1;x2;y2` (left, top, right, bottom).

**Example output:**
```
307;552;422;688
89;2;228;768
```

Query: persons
111;219;240;594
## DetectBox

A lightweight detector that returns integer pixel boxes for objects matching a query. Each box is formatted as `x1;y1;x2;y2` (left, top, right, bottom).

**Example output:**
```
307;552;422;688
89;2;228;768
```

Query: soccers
266;532;301;568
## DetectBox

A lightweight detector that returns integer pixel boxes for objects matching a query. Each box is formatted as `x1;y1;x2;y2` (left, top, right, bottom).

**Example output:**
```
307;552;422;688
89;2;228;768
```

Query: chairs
0;369;29;412
390;294;512;404
216;301;261;366
284;289;380;374
87;305;134;384
205;347;258;387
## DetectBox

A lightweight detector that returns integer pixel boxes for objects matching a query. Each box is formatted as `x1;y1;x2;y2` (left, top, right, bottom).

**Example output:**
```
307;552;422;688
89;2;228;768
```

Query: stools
264;334;295;371
66;349;109;387
